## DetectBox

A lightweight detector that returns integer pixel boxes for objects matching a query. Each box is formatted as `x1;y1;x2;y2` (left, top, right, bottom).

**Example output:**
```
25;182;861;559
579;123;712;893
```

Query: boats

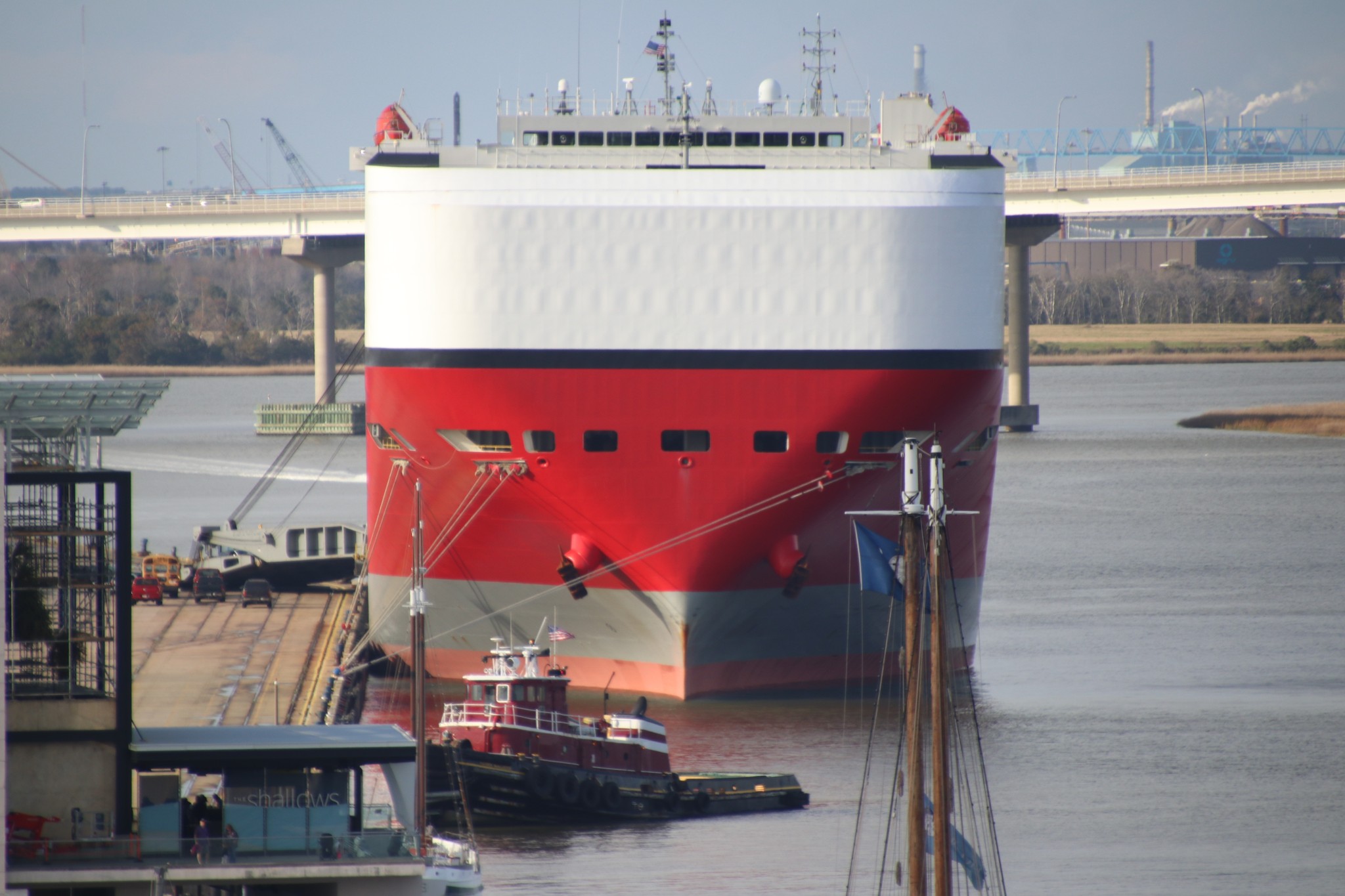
422;835;481;893
421;607;810;831
364;12;1004;699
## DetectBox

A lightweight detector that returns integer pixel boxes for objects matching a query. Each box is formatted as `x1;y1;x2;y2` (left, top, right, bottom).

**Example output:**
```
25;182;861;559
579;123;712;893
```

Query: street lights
1191;88;1208;182
1052;96;1077;187
78;124;101;216
218;118;234;196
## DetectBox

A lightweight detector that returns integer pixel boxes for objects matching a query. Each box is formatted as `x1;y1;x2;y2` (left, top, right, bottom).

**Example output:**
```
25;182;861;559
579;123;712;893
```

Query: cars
131;577;165;606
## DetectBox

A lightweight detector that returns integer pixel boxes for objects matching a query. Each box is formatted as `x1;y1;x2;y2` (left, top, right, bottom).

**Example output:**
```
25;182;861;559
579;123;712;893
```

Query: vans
14;197;44;207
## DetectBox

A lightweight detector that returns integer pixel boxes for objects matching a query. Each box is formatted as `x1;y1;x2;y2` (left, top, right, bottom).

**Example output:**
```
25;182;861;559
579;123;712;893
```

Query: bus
142;554;180;598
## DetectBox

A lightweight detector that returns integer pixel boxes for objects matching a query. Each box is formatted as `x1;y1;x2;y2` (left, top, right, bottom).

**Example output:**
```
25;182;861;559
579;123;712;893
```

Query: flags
547;624;575;642
643;40;665;57
852;520;932;614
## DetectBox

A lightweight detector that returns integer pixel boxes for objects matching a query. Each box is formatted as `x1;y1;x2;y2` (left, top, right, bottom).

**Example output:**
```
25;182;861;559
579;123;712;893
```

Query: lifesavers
600;781;621;811
556;772;580;806
580;778;602;809
694;792;711;814
599;717;608;734
666;791;681;812
524;764;555;800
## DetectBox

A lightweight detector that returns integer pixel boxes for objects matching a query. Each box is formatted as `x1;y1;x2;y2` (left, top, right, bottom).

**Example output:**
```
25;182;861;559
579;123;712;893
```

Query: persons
140;794;221;838
220;823;239;867
386;829;404;857
193;818;213;867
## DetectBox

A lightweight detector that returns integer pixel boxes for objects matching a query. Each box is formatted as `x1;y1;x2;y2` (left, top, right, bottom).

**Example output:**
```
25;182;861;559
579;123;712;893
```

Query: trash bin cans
322;834;332;860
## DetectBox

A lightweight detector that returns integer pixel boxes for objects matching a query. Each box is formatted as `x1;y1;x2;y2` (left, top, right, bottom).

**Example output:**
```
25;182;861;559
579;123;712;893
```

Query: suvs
193;568;226;602
242;577;273;609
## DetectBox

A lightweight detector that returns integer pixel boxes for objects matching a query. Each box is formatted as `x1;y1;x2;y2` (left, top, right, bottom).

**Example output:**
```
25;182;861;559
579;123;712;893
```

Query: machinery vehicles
260;115;321;199
195;115;275;196
